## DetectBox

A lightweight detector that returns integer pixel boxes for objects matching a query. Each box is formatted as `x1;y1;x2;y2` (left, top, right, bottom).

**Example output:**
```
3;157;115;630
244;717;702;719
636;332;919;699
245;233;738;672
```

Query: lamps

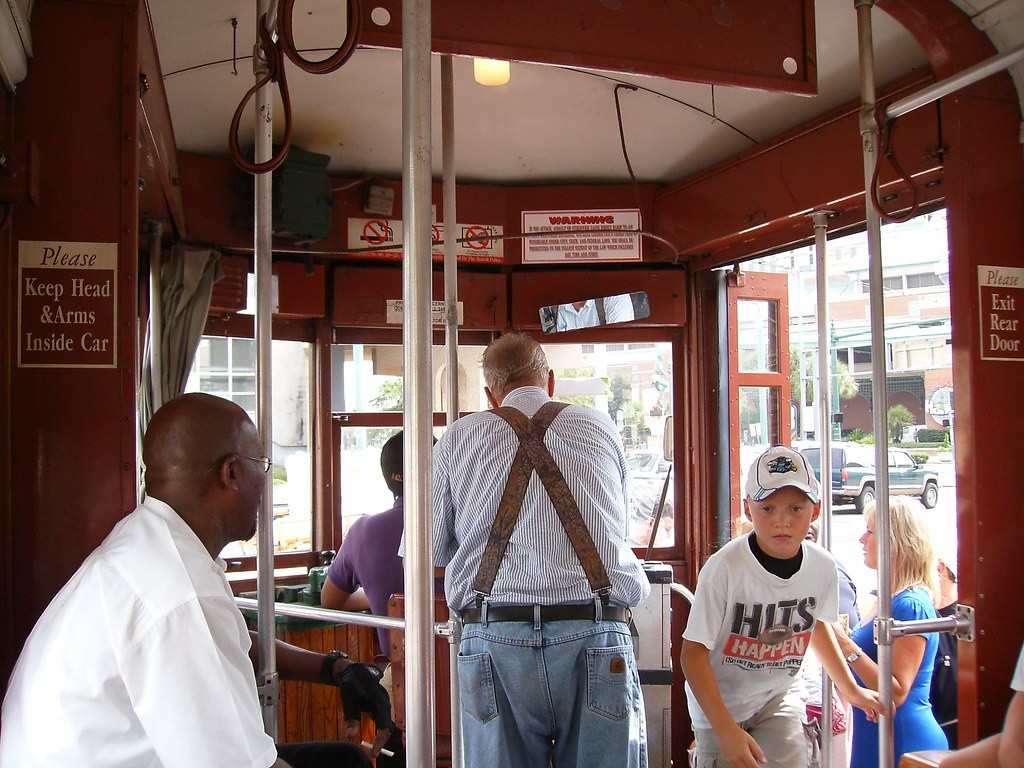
473;56;510;85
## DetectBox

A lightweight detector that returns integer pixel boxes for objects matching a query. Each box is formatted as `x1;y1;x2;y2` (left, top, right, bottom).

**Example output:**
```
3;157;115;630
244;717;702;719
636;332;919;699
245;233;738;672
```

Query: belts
460;604;629;624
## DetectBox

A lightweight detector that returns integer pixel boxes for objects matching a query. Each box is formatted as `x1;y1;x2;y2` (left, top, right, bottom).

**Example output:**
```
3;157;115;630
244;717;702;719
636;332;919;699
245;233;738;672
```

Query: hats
746;444;820;505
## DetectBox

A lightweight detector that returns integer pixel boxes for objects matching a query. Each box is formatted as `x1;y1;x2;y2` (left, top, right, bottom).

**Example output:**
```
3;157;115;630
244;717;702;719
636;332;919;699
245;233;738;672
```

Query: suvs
801;444;940;515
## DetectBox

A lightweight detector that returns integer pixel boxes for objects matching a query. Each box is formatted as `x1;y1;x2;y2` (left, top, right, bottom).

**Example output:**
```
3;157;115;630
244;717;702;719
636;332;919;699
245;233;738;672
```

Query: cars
625;450;674;486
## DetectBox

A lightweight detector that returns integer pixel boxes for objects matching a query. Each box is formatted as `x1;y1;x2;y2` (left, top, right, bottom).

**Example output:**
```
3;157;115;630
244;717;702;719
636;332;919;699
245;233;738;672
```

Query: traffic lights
834;412;844;423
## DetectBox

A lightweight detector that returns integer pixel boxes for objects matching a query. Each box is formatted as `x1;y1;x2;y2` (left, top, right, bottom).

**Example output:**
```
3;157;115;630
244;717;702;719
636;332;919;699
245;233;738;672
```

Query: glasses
204;456;271;475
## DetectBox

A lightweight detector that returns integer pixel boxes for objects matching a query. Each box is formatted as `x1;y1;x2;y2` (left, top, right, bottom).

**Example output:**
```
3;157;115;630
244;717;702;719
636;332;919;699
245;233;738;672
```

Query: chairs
387;592;452;762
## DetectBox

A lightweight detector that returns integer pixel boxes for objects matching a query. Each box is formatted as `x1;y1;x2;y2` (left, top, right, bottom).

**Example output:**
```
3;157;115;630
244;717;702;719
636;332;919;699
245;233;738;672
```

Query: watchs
320;650;350;687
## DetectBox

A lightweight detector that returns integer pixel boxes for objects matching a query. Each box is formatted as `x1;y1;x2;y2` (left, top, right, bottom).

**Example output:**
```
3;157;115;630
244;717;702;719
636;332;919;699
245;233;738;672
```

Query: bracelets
846;647;865;664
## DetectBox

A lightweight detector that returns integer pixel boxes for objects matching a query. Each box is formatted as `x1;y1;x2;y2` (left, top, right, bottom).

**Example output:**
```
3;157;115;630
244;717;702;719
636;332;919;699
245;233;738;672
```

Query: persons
939;636;1024;768
1;391;397;768
395;330;652;768
557;294;634;331
626;443;956;768
320;428;472;662
